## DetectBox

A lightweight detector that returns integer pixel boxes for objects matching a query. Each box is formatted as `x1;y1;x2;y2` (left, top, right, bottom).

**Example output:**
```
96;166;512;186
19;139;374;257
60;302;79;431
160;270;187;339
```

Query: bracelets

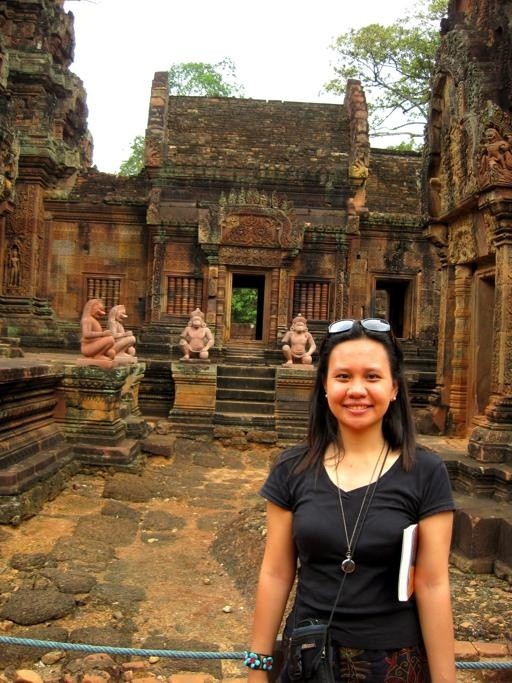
242;651;274;672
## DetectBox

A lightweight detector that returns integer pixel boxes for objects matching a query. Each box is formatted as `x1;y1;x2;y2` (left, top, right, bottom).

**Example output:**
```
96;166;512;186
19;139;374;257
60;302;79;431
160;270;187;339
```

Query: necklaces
332;440;385;573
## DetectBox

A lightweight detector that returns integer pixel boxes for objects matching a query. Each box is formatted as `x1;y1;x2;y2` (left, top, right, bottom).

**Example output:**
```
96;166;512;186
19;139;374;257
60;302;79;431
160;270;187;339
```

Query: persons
280;313;316;367
242;318;458;683
79;299;115;361
106;304;136;360
179;308;214;360
480;129;509;174
9;251;20;287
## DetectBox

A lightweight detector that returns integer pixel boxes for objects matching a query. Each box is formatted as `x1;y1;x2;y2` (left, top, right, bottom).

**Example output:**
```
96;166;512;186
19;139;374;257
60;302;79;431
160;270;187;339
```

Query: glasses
327;318;396;343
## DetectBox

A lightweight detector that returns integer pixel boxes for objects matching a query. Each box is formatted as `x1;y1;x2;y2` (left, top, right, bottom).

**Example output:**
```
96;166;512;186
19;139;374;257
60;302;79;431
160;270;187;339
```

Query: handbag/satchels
289;625;335;683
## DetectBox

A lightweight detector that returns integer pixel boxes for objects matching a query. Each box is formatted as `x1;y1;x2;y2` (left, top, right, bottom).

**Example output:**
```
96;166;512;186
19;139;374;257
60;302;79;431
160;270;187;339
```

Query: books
398;523;420;603
